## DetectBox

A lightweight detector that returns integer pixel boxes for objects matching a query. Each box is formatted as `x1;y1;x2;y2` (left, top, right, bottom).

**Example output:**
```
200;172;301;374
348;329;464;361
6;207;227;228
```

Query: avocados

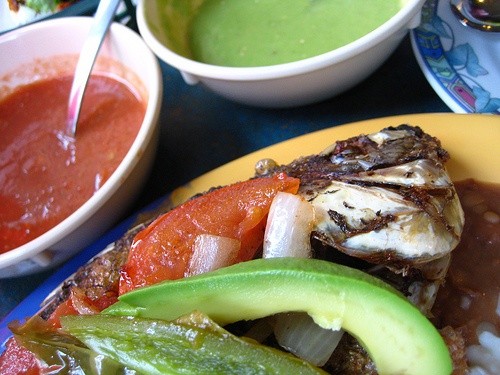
98;256;455;375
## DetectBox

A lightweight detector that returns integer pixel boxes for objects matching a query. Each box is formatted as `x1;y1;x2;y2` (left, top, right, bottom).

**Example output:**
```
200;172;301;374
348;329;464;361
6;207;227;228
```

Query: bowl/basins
135;0;425;110
0;16;165;279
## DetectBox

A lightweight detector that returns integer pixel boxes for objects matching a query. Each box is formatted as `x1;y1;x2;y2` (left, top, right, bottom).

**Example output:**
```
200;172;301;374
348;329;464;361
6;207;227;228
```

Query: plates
0;112;500;375
410;0;500;115
